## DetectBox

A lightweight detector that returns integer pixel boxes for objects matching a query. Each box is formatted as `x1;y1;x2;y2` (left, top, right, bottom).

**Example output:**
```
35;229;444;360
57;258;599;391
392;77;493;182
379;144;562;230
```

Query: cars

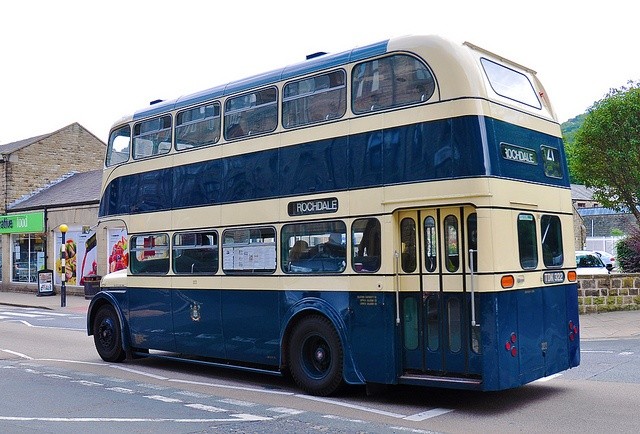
595;251;617;271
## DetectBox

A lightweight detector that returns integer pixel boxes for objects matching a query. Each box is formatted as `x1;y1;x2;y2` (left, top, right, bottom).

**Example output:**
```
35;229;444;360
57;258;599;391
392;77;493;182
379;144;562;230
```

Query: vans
575;250;610;275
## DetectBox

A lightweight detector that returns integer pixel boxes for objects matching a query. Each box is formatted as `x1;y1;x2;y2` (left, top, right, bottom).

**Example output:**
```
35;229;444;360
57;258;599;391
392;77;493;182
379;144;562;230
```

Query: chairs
286;259;379;272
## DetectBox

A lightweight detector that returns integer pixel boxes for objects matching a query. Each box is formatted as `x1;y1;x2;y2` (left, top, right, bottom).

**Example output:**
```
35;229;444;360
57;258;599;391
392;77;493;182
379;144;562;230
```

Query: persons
358;218;381;257
197;236;211;254
312;232;344;256
288;240;308;262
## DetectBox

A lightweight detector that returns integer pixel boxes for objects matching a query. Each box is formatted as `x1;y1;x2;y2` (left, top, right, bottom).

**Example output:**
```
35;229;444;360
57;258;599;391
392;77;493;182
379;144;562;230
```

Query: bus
81;33;580;397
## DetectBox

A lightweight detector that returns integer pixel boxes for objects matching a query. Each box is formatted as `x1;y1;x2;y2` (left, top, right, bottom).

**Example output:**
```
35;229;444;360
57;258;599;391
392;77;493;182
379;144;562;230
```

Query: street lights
58;224;69;307
